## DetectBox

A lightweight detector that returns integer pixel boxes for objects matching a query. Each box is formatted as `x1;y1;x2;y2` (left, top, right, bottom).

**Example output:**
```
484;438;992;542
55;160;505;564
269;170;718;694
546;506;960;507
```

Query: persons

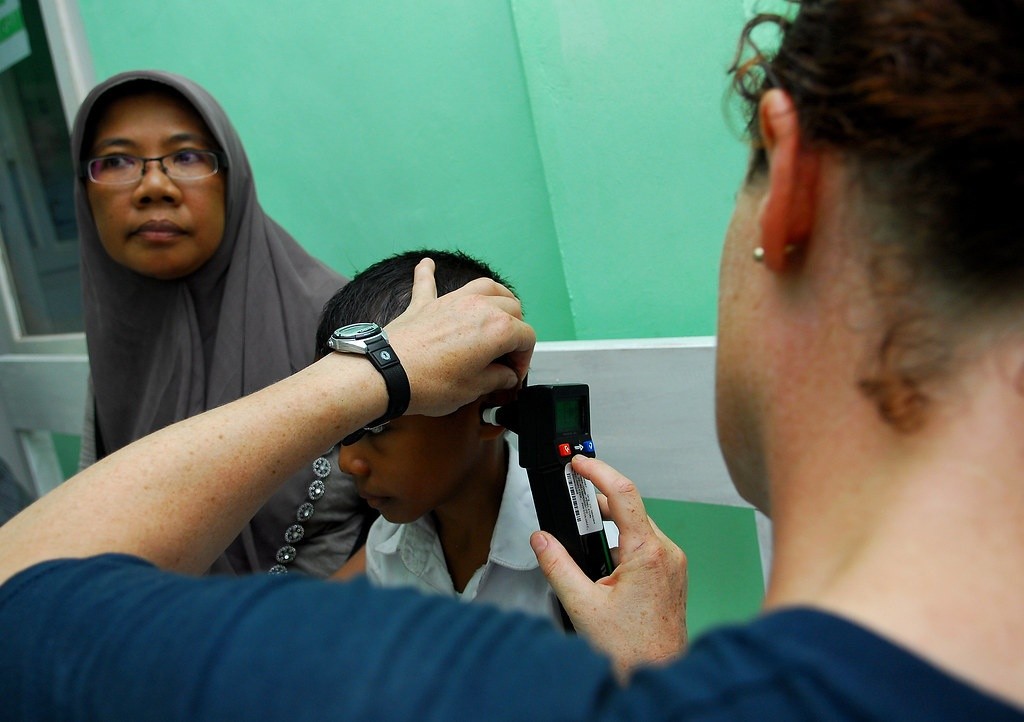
0;1;1024;720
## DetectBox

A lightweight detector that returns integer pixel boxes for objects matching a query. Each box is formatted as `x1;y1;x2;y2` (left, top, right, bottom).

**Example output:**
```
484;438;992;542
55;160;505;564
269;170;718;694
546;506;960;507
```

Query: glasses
88;149;220;185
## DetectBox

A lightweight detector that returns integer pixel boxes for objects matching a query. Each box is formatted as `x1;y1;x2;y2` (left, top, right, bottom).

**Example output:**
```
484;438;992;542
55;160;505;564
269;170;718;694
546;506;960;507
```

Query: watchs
326;322;411;430
